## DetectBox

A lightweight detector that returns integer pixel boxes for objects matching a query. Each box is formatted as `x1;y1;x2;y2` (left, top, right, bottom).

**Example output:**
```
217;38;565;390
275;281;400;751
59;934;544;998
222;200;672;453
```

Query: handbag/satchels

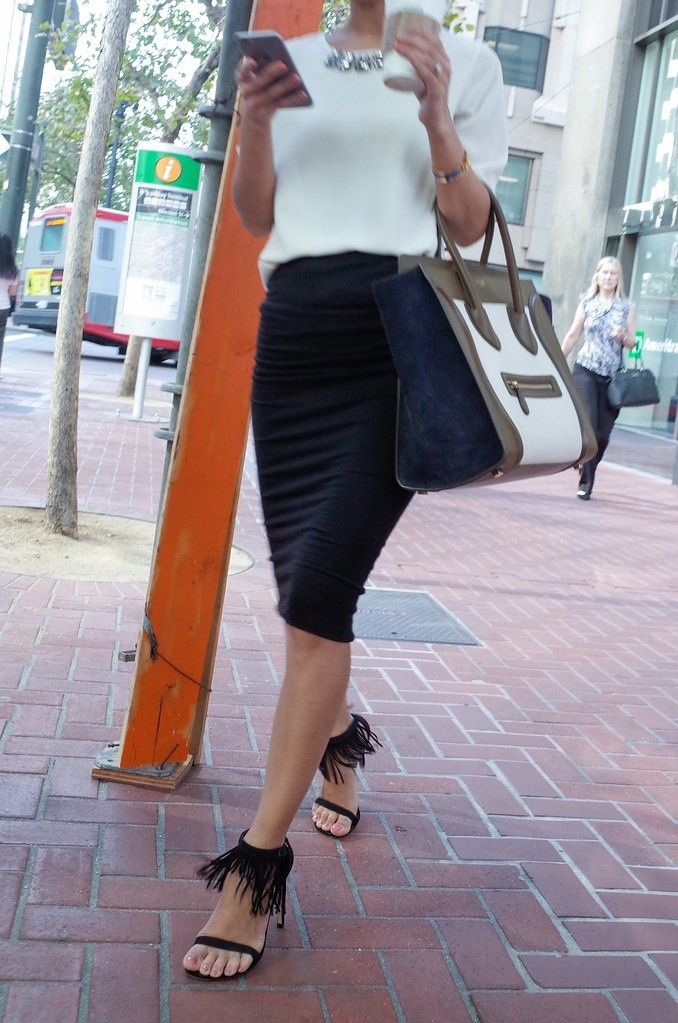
370;171;600;496
606;340;659;408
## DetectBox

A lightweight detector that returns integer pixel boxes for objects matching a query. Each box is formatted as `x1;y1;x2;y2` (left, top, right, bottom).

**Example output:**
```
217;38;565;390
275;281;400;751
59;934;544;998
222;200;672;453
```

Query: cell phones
235;29;312;108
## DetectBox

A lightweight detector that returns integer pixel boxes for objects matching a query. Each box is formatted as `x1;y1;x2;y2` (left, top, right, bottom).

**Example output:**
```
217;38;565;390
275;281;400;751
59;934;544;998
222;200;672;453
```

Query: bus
14;204;182;366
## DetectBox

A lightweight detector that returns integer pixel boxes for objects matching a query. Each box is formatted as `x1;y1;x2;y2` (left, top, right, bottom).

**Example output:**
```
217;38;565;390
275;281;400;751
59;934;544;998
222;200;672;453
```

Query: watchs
621;335;627;342
432;148;470;185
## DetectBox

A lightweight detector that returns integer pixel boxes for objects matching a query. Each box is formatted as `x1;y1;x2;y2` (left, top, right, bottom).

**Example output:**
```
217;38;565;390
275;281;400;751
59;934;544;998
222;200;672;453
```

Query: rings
434;62;443;74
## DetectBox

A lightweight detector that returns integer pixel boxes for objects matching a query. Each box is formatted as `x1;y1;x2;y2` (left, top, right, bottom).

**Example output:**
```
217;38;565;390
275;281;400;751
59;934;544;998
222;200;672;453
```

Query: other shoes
577;490;591;500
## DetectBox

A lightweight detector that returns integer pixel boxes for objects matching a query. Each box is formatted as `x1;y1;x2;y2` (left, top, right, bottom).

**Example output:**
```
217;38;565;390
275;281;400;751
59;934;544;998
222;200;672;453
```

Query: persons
0;232;19;381
561;256;638;500
180;0;511;978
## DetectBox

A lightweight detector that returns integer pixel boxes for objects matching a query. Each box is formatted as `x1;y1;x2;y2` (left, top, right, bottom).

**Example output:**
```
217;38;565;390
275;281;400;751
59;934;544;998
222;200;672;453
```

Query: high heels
181;827;295;981
312;712;386;840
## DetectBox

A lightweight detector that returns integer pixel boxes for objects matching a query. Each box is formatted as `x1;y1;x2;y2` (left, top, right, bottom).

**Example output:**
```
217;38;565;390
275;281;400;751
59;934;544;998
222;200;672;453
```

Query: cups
381;1;447;95
